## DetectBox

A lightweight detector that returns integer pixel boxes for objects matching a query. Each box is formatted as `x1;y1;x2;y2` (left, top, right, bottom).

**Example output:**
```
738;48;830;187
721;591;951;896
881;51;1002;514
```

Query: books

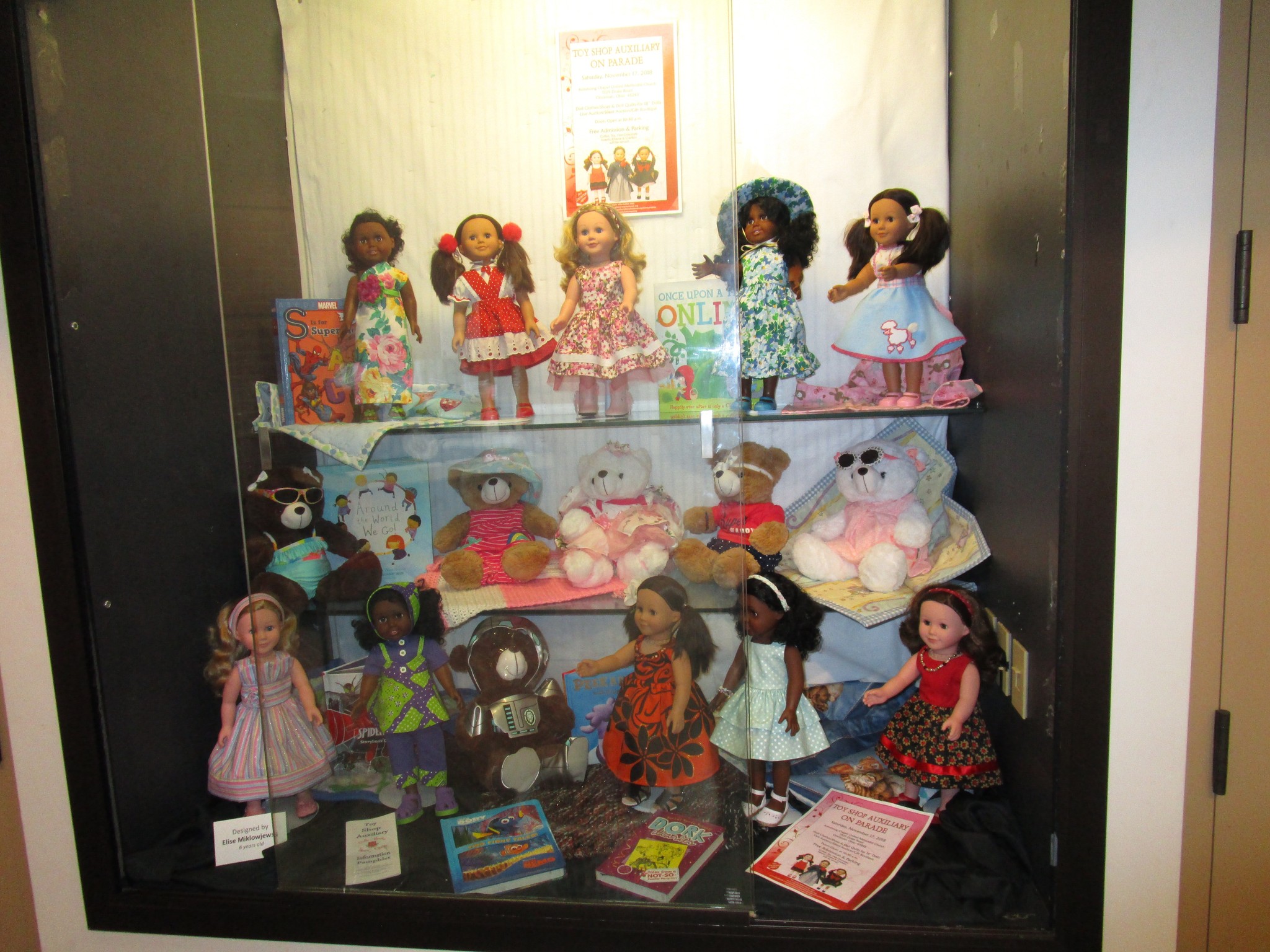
271;295;370;428
322;653;395;779
595;808;727;905
650;276;748;413
439;797;567;897
310;456;432;584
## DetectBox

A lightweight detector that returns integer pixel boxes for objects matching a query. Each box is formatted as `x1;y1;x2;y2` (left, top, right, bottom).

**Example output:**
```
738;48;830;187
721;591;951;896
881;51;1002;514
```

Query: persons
708;569;824;825
345;589;462;822
325;159;969;424
573;575;711;816
193;592;335;830
863;583;1001;826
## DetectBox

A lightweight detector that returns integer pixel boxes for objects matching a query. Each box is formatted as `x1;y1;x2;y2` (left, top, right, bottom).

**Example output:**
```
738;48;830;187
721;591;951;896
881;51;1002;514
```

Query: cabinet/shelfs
236;371;969;781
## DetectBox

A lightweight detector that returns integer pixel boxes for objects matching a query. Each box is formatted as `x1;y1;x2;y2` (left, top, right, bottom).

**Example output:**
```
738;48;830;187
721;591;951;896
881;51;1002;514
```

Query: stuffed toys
679;439;793;587
234;463;385;613
559;442;684;591
430;447;559;591
792;436;936;588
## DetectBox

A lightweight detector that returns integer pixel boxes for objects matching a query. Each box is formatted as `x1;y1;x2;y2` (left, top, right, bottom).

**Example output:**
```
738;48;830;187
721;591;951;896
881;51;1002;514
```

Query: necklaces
918;645;961;672
635;634;670;662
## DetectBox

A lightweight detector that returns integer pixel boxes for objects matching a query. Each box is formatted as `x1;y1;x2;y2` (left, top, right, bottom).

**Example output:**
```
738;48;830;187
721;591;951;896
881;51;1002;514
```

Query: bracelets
717;686;735;699
